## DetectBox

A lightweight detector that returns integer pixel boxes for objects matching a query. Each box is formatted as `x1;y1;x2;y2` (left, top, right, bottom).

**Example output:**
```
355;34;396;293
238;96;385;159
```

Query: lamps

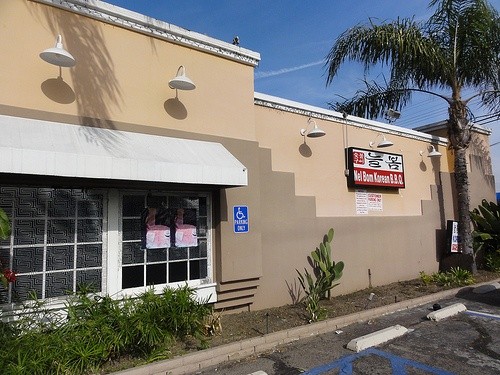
40;34;76;67
168;65;197;90
419;144;442;157
300;120;326;138
369;133;393;148
